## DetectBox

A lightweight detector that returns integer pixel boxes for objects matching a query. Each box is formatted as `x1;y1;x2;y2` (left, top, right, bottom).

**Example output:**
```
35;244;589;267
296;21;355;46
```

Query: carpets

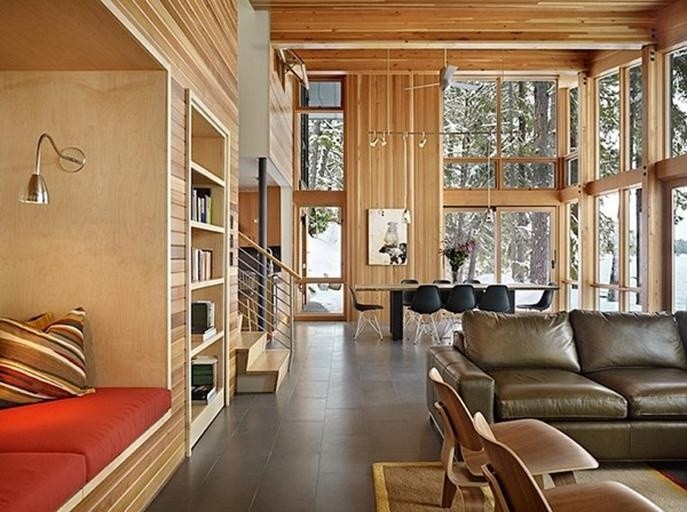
372;462;687;512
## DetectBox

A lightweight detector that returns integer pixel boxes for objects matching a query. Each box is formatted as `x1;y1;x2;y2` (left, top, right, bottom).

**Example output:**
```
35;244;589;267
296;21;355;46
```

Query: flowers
437;236;476;271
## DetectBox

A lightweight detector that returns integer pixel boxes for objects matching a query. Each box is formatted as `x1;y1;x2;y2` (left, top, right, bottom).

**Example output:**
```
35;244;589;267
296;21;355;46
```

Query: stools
239;298;258;332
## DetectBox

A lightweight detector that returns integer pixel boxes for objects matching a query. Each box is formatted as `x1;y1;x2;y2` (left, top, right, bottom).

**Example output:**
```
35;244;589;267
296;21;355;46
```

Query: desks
354;284;560;341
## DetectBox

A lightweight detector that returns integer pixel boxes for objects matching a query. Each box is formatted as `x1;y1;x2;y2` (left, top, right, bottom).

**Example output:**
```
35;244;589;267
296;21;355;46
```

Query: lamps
439;50;459;91
18;133;86;205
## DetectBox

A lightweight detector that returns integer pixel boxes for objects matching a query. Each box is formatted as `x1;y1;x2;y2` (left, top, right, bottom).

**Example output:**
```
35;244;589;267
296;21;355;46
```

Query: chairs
440;284;477;344
480;463;515;512
433;400;488;512
463;279;484;309
349;287;384;341
517;282;557;312
401;279;429;331
431;279;454;328
473;411;663;512
429;367;600;512
479;285;511;312
403;285;442;344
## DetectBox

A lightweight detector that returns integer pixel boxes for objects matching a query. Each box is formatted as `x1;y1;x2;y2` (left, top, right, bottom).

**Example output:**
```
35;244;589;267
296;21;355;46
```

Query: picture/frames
367;208;411;266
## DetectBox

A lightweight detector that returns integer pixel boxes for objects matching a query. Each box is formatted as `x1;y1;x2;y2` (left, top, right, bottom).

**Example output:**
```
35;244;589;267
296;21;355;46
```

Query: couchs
1;386;174;512
426;310;687;463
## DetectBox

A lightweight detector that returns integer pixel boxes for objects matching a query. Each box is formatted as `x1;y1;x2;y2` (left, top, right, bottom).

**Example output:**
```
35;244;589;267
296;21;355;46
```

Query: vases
452;266;459;283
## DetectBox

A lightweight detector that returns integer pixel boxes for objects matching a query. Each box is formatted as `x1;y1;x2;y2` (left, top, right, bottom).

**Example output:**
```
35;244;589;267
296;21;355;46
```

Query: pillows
0;306;97;405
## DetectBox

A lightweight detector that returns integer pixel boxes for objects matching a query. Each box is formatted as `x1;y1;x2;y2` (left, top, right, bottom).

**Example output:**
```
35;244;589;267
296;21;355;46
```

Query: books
190;245;214;284
191;299;217;342
191;354;218;405
191;188;212;225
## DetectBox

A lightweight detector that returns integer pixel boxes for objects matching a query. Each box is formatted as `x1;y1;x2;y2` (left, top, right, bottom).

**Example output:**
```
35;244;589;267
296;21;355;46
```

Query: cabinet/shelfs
185;88;231;458
